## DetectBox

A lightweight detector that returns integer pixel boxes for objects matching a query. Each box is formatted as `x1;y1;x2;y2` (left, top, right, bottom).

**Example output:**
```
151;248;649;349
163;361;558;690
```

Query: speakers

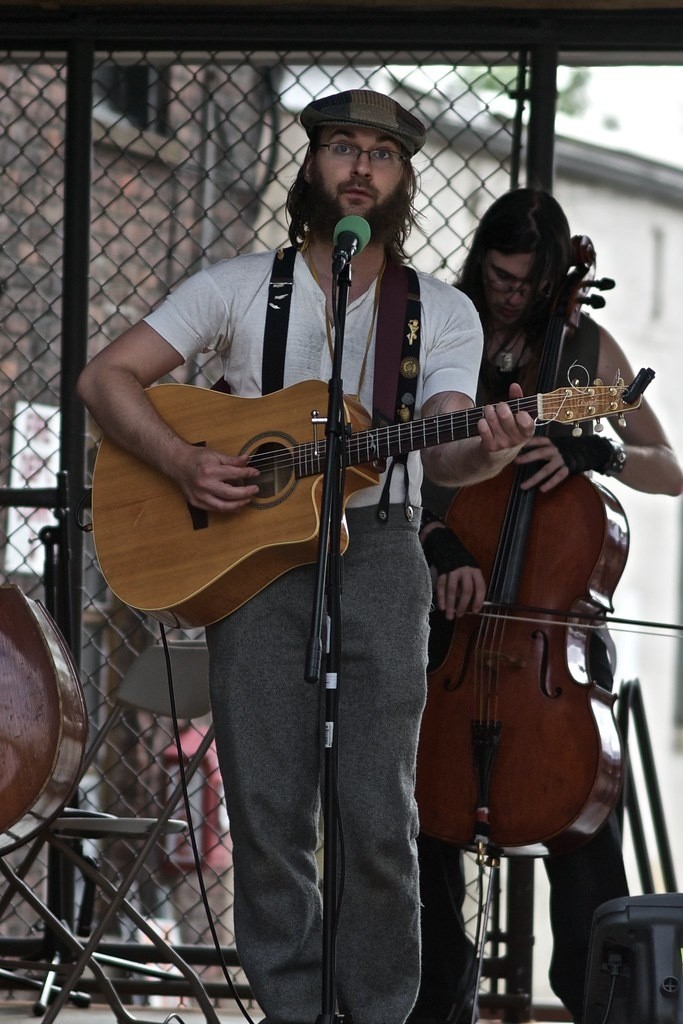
584;893;683;1024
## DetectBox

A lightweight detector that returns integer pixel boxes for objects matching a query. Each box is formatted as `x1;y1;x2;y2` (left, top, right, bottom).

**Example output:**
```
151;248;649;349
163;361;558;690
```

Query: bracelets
606;435;626;477
419;509;445;531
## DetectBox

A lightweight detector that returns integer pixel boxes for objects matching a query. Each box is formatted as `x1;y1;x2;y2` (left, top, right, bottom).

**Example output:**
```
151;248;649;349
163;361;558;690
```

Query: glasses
316;143;405;164
480;263;548;298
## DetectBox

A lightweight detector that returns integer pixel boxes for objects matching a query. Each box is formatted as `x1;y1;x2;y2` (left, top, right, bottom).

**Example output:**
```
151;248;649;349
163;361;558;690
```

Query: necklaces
305;245;384;395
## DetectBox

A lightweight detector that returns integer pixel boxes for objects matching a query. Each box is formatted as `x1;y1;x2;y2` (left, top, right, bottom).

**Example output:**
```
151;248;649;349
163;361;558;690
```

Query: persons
406;189;683;1024
77;89;535;1024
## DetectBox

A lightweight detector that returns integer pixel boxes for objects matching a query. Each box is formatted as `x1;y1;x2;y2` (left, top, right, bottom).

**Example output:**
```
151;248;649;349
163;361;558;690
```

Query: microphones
331;216;372;276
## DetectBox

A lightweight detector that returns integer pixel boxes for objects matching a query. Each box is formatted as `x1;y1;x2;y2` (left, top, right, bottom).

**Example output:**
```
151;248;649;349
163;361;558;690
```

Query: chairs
0;638;241;1024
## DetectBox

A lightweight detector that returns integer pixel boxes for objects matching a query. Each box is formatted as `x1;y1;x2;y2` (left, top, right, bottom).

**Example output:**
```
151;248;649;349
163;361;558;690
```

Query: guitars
92;369;659;632
0;578;88;855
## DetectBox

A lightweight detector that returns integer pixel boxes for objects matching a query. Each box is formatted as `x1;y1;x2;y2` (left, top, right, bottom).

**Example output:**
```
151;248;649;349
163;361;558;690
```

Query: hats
300;90;425;157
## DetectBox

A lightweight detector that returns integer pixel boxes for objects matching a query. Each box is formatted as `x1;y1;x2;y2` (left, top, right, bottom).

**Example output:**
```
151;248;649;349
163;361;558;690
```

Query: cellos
415;236;627;859
